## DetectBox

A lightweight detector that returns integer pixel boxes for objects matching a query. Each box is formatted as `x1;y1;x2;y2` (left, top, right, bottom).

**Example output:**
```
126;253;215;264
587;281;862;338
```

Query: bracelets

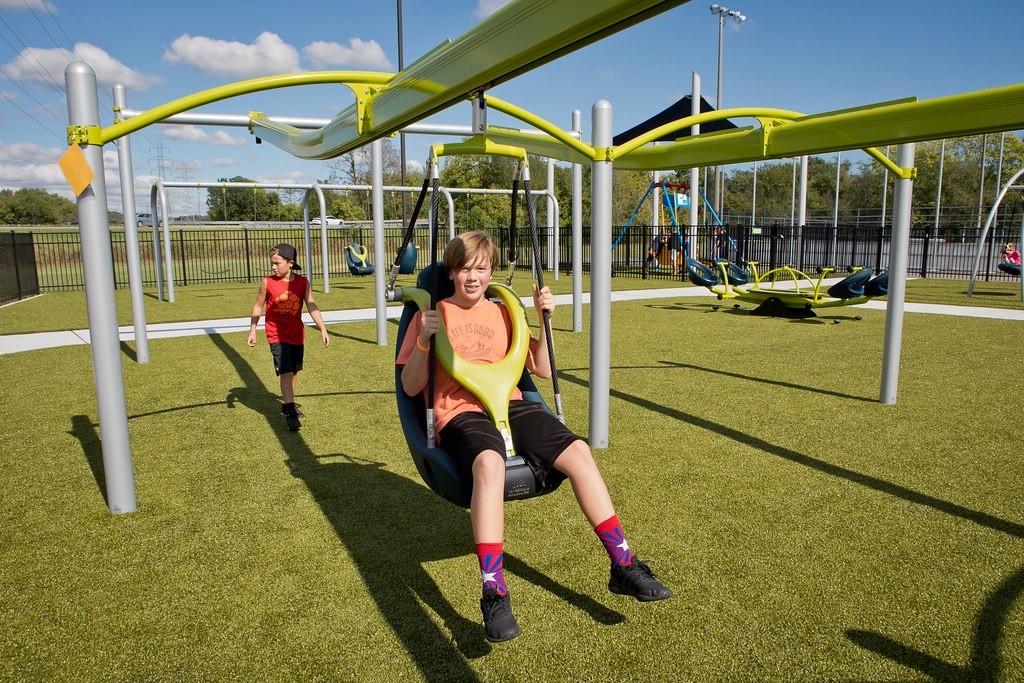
417;335;430;353
249;334;256;338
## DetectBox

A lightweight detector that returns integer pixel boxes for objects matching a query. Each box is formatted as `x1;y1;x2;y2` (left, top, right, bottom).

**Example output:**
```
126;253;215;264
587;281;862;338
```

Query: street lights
710;3;747;111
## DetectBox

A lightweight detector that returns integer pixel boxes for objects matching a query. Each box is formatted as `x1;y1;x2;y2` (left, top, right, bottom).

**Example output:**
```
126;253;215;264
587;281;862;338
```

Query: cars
136;212;163;227
312;215;344;225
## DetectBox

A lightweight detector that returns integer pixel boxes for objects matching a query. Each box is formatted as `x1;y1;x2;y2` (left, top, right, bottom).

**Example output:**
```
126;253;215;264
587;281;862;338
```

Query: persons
247;243;330;431
999;242;1021;266
397;231;672;643
649;224;727;276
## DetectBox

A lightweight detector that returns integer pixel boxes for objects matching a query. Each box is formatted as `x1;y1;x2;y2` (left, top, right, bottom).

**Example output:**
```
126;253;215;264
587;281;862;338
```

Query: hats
269;243;302;270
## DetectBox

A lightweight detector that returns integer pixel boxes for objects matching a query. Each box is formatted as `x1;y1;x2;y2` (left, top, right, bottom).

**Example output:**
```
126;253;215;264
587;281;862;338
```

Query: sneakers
286;413;302;433
608;554;673;602
479;587;522;643
281;402;305;419
